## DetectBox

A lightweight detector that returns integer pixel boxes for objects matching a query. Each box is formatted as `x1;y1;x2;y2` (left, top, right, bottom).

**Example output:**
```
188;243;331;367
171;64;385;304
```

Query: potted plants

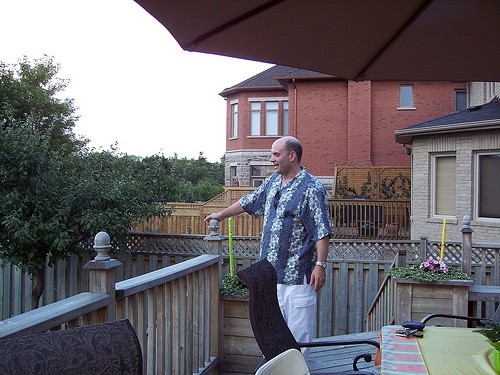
219;273;264;374
329;171;379;237
380;171;411;236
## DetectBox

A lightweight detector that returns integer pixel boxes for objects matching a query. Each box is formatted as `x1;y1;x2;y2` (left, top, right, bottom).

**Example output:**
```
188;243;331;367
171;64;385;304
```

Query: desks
374;325;500;375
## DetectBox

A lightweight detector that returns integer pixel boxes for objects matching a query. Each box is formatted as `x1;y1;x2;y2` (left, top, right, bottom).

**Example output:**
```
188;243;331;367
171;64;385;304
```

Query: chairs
235;258;381;375
0;318;143;375
255;348;310;375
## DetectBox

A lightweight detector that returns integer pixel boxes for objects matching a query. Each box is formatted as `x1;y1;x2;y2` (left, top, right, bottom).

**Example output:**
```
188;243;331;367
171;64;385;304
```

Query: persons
204;134;332;363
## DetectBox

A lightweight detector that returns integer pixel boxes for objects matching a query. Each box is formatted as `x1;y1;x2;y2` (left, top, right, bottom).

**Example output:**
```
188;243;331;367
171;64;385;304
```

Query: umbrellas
133;0;500;88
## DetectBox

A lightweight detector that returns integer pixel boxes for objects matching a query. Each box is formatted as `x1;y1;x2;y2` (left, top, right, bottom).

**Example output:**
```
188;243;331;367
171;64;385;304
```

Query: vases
391;275;473;328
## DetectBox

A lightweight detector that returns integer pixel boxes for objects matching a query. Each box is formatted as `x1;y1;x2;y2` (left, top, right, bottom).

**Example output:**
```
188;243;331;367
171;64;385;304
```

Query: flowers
384;253;473;282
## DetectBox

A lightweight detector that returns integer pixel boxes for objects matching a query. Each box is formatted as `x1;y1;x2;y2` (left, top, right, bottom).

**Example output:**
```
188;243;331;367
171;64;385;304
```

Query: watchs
315;262;327;269
